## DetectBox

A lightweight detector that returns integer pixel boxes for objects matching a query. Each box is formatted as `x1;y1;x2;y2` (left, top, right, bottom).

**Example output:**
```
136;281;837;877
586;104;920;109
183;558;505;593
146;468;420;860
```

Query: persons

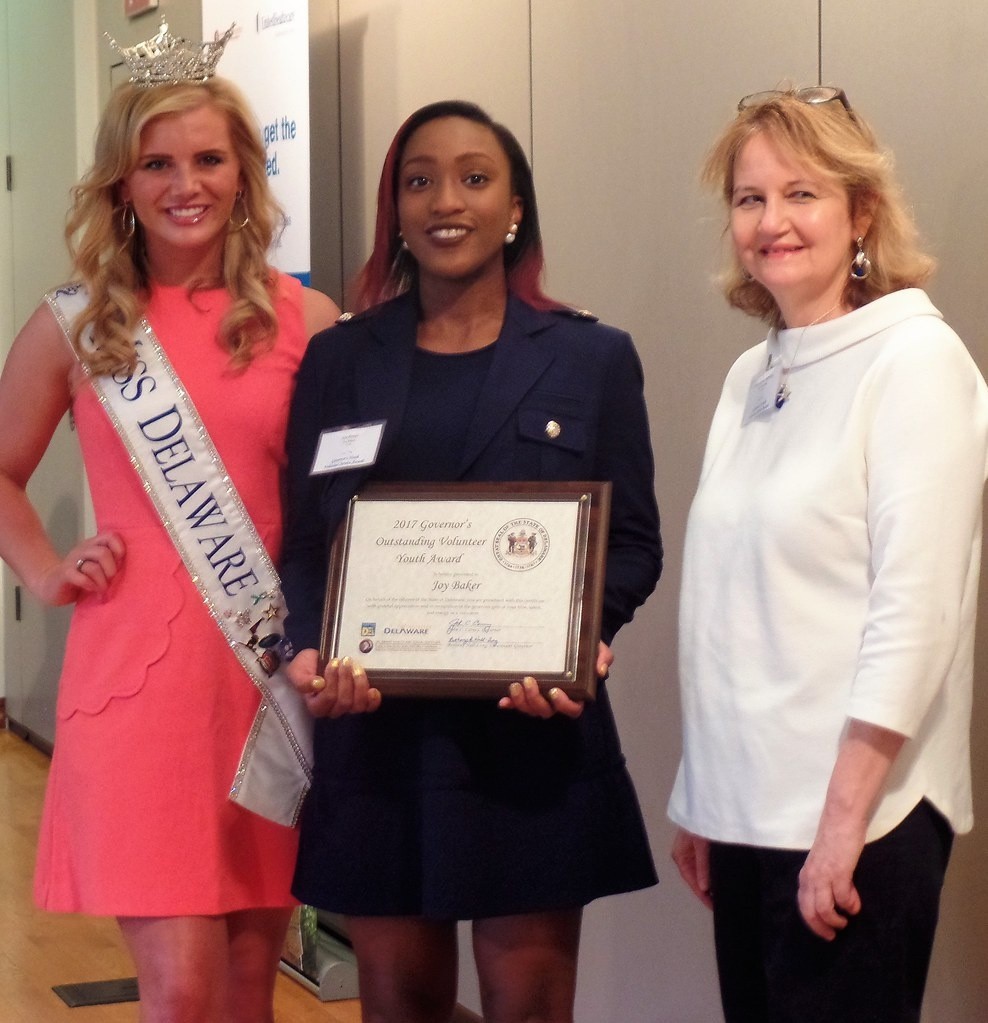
278;97;661;1023
662;72;988;1023
0;8;346;1023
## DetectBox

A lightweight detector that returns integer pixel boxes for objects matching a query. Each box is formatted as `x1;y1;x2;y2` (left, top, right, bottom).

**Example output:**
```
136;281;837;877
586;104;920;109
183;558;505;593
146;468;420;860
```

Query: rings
76;559;84;569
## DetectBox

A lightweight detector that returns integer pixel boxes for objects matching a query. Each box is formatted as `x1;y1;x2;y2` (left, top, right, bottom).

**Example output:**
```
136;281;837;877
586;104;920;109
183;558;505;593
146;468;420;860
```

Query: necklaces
774;301;845;409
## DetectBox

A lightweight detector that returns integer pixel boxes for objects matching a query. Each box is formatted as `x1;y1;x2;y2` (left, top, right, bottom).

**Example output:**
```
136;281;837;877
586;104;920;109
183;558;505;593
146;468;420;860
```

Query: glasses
739;85;861;133
